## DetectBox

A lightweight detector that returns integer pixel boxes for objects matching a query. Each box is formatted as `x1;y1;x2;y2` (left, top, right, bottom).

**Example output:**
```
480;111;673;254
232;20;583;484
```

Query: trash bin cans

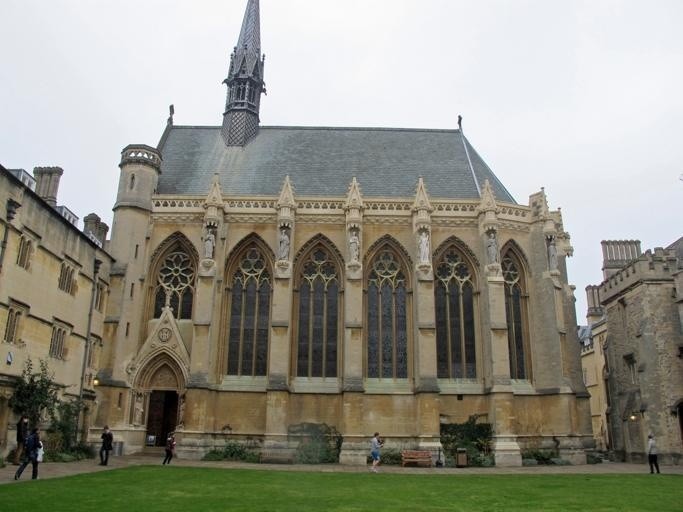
455;448;467;467
112;442;122;456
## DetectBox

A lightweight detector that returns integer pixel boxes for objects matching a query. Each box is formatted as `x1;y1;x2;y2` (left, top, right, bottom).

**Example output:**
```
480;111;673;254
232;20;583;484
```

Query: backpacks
24;433;38;452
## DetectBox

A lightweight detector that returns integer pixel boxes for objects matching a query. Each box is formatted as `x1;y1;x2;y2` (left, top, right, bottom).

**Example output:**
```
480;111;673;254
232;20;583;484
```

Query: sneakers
98;462;108;467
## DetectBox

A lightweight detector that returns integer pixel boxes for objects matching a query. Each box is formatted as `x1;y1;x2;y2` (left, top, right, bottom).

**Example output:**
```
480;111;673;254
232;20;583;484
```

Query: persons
347;230;360;262
98;424;113;466
278;229;289;262
486;233;499;266
162;431;176;466
203;228;216;259
11;414;29;466
368;431;384;473
13;426;40;479
546;240;559;273
416;232;430;262
645;432;660;473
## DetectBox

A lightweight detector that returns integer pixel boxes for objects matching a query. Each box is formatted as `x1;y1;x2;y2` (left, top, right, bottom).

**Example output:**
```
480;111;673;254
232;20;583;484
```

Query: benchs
400;450;432;468
